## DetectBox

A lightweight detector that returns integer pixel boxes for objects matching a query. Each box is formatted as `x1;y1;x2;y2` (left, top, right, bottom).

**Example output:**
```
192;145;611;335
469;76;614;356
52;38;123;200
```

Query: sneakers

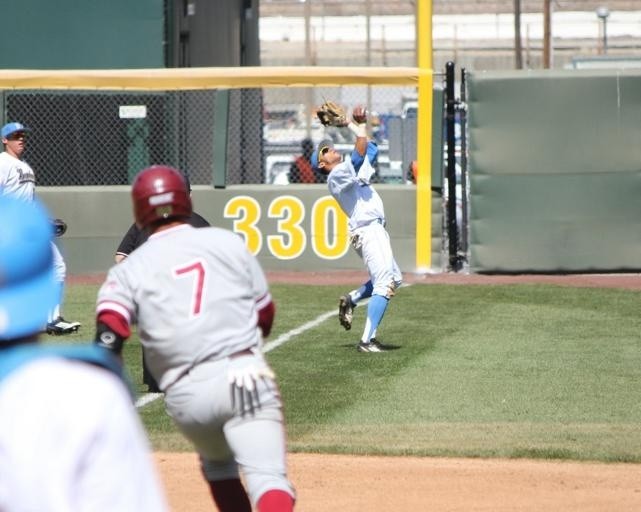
338;293;357;330
45;316;81;336
356;337;388;352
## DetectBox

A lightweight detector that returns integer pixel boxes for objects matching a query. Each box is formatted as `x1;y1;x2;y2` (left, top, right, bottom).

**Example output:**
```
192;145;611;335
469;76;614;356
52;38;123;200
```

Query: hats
0;186;63;340
310;139;336;175
0;120;31;138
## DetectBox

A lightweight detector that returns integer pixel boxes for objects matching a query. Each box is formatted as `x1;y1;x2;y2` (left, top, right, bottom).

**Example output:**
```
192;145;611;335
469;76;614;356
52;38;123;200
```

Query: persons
1;119;82;337
0;186;170;511
93;164;298;512
288;139;327;185
312;101;404;354
114;170;214;396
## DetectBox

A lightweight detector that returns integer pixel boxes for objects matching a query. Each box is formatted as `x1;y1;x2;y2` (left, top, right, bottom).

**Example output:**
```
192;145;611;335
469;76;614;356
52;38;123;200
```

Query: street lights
596;5;609;56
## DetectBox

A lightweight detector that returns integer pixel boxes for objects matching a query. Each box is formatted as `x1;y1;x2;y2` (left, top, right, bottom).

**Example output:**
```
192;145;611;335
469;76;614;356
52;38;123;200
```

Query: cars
263;101;469;233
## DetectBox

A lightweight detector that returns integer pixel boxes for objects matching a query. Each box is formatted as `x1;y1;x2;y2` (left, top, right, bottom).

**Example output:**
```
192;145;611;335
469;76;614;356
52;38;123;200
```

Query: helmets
130;162;193;231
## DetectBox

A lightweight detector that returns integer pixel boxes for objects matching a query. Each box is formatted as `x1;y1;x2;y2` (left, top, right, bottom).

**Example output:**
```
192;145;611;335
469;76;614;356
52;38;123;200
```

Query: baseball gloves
317;102;351;127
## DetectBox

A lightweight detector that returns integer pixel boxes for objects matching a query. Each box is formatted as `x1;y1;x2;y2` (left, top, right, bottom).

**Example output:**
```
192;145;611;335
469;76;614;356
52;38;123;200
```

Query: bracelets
347;123;368;139
359;124;368;129
